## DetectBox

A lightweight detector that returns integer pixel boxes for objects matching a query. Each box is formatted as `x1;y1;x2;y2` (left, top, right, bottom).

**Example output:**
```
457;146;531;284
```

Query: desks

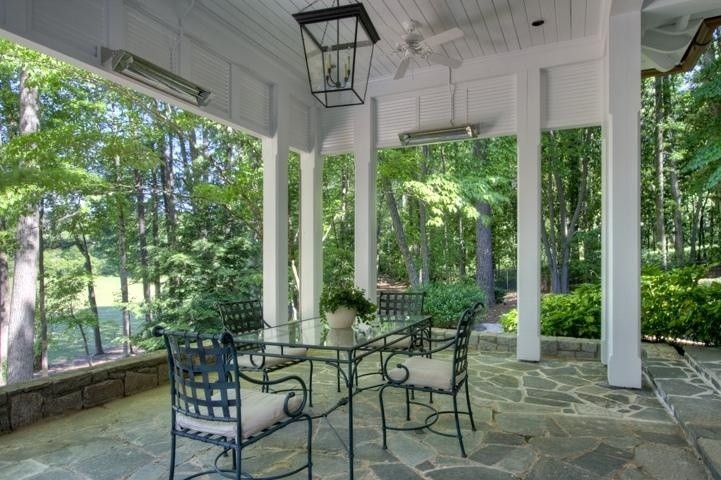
232;316;431;480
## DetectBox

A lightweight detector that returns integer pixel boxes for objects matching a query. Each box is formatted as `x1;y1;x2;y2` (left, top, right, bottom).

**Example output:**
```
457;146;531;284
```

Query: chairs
151;299;315;480
366;291;486;459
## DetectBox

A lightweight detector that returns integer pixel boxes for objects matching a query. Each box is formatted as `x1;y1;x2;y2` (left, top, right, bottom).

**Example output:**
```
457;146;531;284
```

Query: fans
392;18;466;80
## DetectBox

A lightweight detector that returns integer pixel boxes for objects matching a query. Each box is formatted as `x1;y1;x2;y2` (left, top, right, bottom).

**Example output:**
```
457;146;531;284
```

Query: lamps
398;125;475;146
101;45;216;107
291;1;381;110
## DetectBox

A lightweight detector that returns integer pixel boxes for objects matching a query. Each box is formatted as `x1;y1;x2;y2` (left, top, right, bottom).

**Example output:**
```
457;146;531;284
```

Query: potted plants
319;278;378;330
